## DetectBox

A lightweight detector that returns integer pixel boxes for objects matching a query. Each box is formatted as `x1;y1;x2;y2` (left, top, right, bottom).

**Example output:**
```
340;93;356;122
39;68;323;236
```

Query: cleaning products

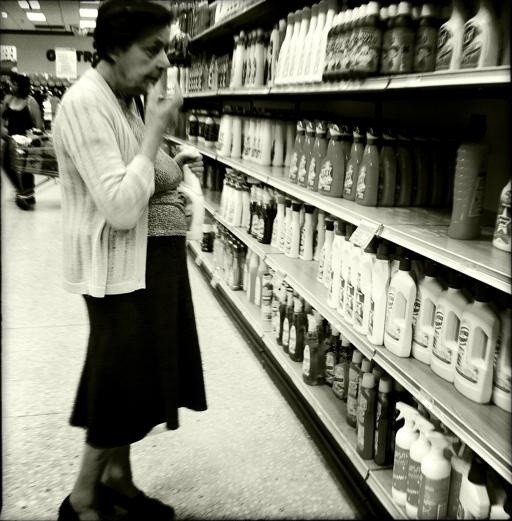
170;0;511;521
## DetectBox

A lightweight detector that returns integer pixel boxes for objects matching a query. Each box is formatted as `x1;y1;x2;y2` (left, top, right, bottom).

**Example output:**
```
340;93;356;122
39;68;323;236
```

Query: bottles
322;1;436;82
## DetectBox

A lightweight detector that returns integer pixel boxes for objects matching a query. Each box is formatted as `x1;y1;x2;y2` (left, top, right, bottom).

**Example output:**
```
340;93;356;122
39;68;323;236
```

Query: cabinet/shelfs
161;0;511;521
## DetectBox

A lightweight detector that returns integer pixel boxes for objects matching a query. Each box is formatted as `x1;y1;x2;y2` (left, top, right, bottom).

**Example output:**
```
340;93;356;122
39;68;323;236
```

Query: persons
0;75;43;209
51;0;208;521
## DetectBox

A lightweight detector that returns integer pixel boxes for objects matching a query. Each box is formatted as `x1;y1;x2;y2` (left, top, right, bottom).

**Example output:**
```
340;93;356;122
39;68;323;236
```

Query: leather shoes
94;477;175;520
57;494;81;520
